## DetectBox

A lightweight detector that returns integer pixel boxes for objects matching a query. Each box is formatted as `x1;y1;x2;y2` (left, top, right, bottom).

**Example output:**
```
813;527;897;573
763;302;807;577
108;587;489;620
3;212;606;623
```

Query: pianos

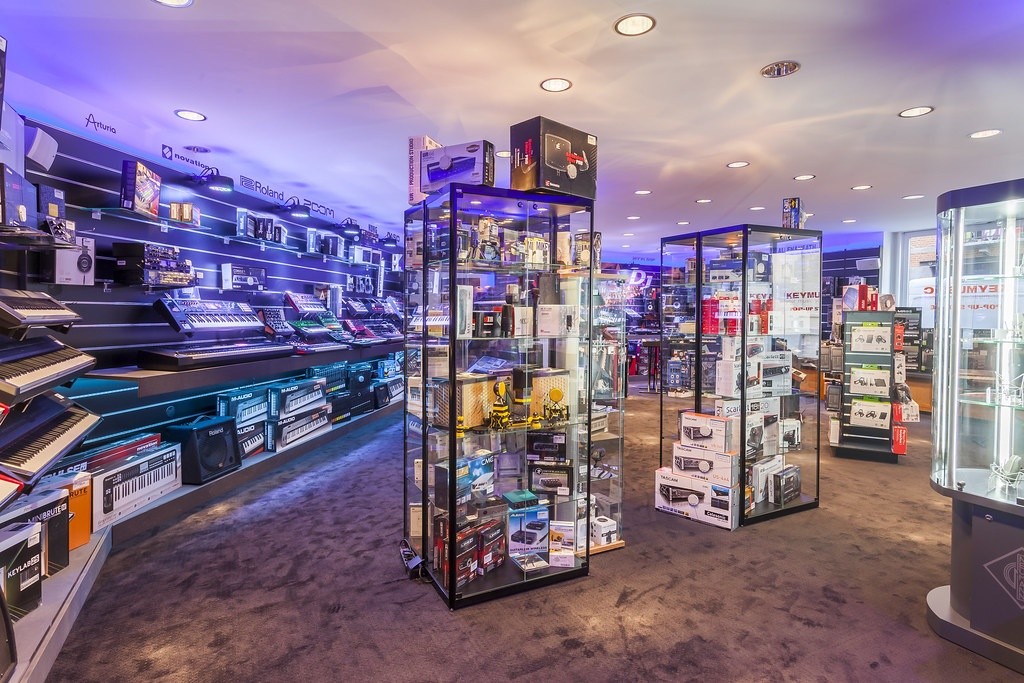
381;376;405;397
92;441;182;534
215;371;333;457
155;298;266;335
1;390;104;485
0;334;98;406
0;289;84;325
137;338;297;371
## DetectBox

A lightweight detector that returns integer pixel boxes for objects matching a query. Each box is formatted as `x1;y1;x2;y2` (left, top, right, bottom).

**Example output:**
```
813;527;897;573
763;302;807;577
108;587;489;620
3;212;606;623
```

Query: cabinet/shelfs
923;176;1023;675
0;37;405;682
654;224;825;532
829;298;907;464
559;272;627;561
401;181;594;611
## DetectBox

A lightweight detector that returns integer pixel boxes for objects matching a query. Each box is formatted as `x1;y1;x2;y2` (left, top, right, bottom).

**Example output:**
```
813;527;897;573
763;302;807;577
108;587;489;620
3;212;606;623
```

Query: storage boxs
890;425;907;455
407;220;601;589
893;352;907;386
418;139;498;194
849;397;892;430
849;362;890;397
507;116;600;199
893;400;920;422
830;344;844;373
577;342;622;554
0;98;402;680
849;320;894;353
828;417;840;445
826;383;841;410
407;132;443;206
893;320;905;350
781;196;804;233
655;253;803;532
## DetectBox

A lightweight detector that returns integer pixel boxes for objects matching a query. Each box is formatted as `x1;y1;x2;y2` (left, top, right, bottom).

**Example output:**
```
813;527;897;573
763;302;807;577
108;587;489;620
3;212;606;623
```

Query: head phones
77;238;92;273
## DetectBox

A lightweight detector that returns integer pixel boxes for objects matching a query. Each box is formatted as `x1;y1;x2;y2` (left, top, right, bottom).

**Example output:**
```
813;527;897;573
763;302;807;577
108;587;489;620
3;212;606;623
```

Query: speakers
24;124;59;172
166;415;242;485
